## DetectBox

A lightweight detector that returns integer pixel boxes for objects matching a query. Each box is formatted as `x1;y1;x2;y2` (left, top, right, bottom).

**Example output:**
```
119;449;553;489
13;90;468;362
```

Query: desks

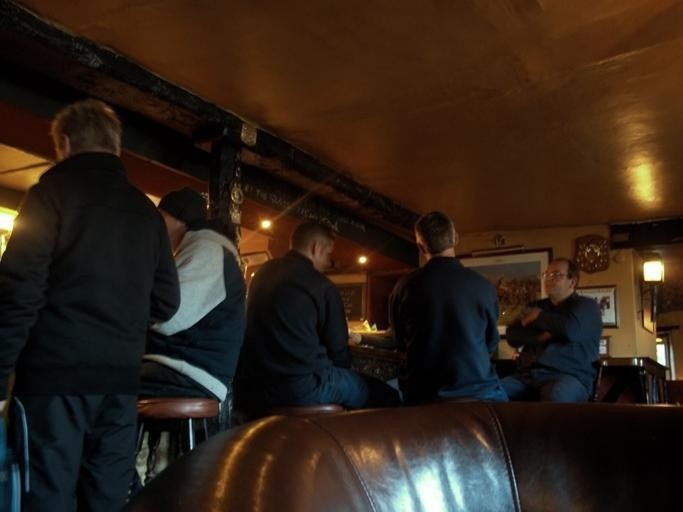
593;356;670;403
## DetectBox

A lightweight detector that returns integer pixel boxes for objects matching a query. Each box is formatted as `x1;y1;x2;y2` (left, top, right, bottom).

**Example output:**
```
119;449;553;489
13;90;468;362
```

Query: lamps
638;252;665;320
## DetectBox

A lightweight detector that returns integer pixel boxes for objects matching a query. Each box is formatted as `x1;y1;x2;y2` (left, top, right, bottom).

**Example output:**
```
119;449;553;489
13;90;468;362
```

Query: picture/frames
577;284;619;329
640;275;655;333
601;336;610;357
335;282;367;323
367;269;409;322
457;247;554;339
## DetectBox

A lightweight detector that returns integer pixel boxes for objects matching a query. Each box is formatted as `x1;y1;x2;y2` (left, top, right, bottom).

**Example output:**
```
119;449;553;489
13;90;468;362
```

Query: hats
159;187;206;226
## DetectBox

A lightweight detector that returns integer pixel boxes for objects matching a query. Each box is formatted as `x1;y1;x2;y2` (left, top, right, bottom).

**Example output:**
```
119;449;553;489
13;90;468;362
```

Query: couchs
127;398;683;512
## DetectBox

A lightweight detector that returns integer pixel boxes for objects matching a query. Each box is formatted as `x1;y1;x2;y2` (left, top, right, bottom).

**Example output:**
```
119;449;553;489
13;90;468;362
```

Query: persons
233;217;401;410
137;185;246;400
1;98;182;512
388;209;509;407
501;258;603;400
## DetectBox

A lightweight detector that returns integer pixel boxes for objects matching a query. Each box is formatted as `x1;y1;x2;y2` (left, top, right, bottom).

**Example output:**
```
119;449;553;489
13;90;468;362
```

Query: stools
124;398;220;505
269;404;342;416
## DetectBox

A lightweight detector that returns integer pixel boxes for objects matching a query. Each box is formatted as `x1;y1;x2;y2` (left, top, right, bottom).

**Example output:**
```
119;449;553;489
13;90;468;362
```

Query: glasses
543;271;567;278
